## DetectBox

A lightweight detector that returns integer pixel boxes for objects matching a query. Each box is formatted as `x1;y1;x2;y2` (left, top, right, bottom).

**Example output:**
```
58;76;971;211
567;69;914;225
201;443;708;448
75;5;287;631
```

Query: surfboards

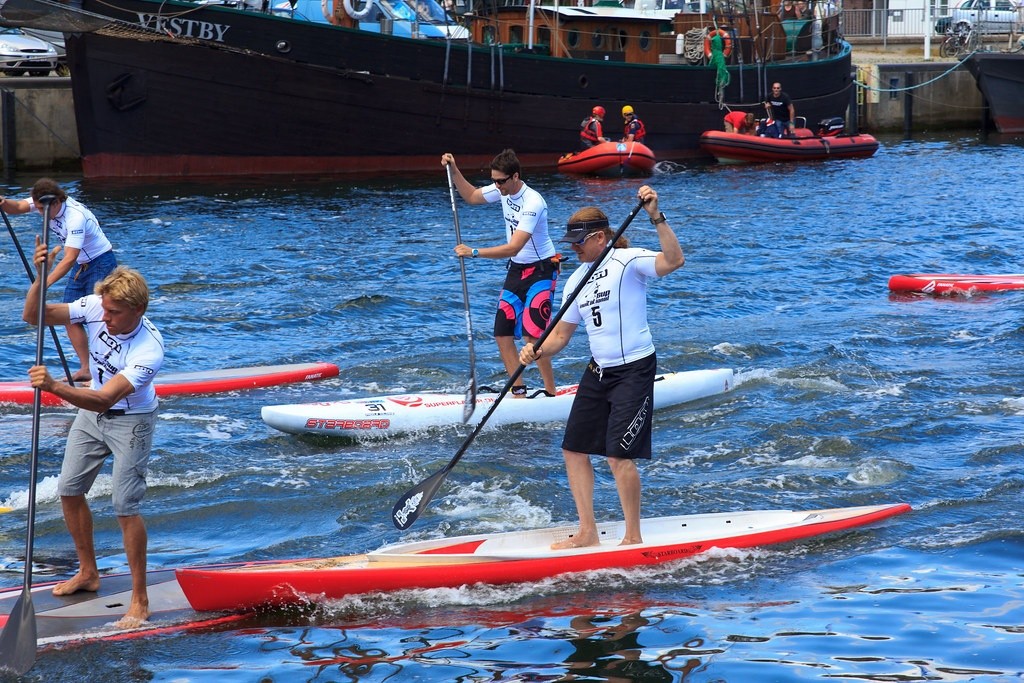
888;270;1024;293
173;503;911;612
0;363;339;405
260;366;734;437
0;559;327;651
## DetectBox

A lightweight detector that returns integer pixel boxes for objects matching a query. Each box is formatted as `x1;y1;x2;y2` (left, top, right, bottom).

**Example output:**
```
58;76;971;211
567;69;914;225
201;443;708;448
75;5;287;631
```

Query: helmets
622;105;634;118
592;106;606;119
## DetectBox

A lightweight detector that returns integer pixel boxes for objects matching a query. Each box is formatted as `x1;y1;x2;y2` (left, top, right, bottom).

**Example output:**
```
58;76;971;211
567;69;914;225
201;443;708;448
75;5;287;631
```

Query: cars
951;0;1021;35
269;0;446;42
1;25;57;78
935;16;952;37
386;0;472;44
20;26;70;76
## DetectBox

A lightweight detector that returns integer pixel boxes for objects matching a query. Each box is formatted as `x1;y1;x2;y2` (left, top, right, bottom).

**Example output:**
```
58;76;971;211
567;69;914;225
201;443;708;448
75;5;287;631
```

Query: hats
558;220;609;244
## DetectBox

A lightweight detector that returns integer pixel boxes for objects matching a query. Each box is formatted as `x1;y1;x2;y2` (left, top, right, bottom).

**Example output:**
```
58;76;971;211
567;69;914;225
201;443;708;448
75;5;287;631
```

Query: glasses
625;113;633;116
490;172;515;185
575;232;598;246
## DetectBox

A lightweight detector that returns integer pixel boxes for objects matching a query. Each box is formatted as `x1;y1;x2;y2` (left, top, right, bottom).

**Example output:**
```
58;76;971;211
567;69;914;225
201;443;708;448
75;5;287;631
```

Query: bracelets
790;122;794;125
534;347;543;360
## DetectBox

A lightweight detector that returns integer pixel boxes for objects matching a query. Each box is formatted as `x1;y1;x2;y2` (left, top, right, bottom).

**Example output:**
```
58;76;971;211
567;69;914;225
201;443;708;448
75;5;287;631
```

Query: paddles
0;194;57;674
445;155;476;424
392;197;653;530
1;198;74;386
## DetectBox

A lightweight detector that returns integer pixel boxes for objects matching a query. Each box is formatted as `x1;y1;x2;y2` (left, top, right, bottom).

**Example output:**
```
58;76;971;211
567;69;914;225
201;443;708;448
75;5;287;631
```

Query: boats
0;0;860;186
955;35;1024;134
697;113;881;165
558;136;658;177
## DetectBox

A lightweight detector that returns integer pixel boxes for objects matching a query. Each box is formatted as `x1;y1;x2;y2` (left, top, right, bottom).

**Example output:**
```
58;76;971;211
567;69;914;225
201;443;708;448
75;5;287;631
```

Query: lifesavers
704;29;732;58
343;0;373;19
322;0;333;22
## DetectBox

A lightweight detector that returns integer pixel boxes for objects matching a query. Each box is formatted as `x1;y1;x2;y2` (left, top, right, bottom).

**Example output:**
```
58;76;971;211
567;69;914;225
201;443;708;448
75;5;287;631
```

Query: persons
441;150;560;399
519;185;686;551
781;0;813;20
724;111;757;136
620;106;646;144
577;106;608;152
22;235;164;630
0;179;118;381
766;84;796;130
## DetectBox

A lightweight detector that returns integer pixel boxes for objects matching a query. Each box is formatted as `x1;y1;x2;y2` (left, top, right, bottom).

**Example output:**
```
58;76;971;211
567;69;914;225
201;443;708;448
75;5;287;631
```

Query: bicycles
939;22;985;58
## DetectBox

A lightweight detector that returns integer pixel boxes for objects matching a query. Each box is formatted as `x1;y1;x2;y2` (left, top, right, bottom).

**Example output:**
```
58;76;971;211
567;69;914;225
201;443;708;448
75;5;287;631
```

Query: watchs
472;248;478;258
650;212;666;224
